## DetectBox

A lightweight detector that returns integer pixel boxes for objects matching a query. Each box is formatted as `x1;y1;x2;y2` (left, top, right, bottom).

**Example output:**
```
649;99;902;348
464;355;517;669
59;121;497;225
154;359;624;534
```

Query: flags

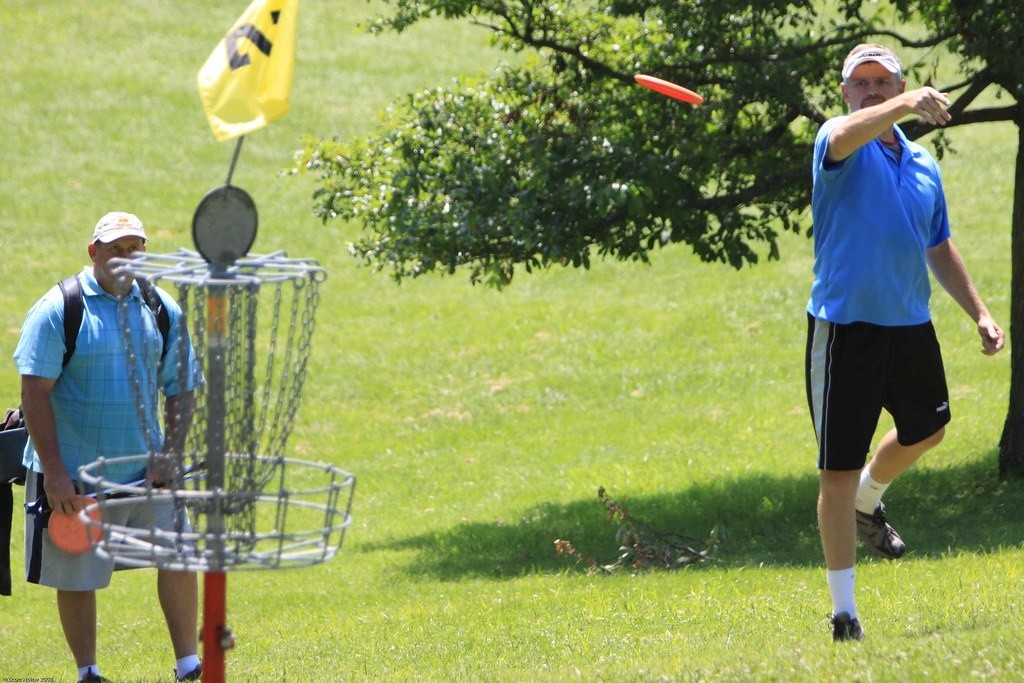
197;0;298;143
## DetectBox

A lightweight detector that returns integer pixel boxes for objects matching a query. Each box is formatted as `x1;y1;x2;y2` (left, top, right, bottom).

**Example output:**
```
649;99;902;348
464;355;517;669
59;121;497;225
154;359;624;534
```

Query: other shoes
78;667;109;683
173;663;204;682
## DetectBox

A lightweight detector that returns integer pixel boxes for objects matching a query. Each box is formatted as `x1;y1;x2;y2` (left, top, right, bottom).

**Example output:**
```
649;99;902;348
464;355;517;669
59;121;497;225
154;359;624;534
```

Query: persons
10;212;206;682
806;45;1003;643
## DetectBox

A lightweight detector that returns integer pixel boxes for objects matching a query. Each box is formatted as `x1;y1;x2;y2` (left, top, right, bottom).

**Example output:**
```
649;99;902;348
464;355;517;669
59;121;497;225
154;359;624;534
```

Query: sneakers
827;611;866;642
855;502;905;558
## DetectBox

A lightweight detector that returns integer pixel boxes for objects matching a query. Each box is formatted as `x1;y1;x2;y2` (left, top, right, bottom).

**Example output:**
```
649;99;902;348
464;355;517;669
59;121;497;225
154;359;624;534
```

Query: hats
842;48;901;80
93;212;148;243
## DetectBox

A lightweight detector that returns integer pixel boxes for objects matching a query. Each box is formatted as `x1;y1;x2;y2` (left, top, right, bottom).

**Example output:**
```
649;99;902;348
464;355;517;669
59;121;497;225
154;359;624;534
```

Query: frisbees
634;73;705;105
48;494;104;555
